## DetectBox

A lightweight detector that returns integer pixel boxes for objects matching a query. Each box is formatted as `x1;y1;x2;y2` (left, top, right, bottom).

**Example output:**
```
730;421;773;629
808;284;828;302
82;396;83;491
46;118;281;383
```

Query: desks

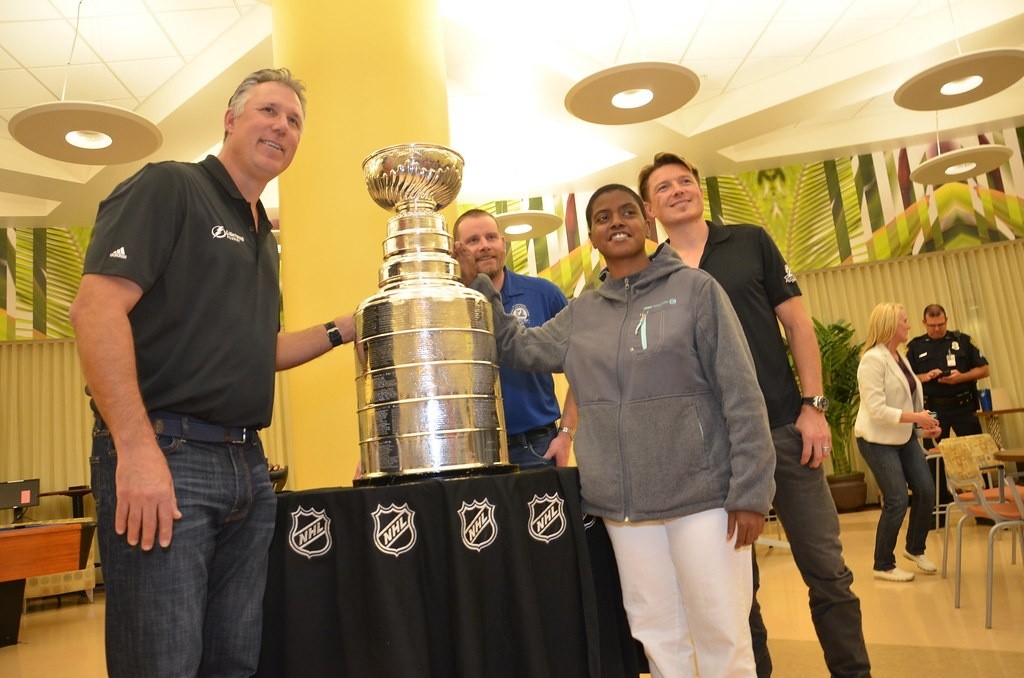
0;520;99;648
37;486;92;598
972;407;1024;451
253;467;650;678
992;447;1024;487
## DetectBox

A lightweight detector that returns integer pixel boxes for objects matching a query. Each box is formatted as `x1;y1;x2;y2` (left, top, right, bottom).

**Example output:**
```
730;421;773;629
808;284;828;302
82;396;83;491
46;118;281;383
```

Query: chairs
917;432;1024;630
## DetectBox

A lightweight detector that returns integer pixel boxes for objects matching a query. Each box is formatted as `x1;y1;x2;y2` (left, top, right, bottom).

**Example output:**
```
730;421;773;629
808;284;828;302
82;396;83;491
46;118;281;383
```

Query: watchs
802;396;830;413
558;427;576;440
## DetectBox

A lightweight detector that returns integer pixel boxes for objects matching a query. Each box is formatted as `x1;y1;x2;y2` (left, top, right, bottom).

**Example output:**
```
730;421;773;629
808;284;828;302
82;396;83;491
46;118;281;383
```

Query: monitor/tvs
0;478;40;523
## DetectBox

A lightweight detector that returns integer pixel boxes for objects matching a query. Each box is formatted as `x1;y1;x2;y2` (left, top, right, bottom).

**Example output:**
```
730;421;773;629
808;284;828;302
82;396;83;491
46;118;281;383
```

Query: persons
638;152;873;678
453;183;776;677
905;304;996;530
853;301;941;582
453;209;578;469
71;69;358;678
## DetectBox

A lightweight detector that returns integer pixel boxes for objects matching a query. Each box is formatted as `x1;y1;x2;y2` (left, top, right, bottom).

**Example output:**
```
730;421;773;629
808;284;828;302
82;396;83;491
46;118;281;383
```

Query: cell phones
939;371;952;378
914;412;938;429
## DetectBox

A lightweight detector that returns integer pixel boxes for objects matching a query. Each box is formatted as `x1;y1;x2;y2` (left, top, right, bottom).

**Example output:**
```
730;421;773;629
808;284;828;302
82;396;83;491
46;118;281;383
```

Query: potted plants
783;317;872;514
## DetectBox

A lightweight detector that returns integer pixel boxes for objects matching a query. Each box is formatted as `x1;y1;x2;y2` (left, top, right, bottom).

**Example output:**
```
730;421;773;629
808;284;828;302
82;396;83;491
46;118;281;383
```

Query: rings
822;447;830;451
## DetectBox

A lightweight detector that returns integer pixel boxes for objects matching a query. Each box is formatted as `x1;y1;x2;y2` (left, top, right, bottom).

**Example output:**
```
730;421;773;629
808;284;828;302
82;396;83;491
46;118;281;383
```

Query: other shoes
976;517;996;526
903;548;937;573
873;565;915;582
929;517;945;530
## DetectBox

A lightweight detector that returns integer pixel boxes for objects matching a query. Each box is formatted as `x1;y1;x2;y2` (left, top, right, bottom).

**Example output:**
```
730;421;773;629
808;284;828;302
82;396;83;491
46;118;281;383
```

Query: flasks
977;388;992;413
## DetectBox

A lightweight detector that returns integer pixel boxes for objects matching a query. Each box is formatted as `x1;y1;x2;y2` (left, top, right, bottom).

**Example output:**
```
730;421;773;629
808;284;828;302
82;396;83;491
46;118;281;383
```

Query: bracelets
324;321;343;348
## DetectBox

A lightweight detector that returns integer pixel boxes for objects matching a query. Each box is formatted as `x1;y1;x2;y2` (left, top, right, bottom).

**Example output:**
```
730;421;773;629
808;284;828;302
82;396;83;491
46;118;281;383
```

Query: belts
506;422;556;446
148;417;258;443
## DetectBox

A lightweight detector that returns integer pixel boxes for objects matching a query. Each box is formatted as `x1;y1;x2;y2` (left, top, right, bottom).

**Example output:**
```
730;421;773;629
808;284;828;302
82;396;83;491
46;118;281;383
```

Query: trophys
352;144;520;486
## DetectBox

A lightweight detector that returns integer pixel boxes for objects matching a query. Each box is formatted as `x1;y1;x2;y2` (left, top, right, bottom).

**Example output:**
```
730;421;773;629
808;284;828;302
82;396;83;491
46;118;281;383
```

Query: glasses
924;321;947;329
